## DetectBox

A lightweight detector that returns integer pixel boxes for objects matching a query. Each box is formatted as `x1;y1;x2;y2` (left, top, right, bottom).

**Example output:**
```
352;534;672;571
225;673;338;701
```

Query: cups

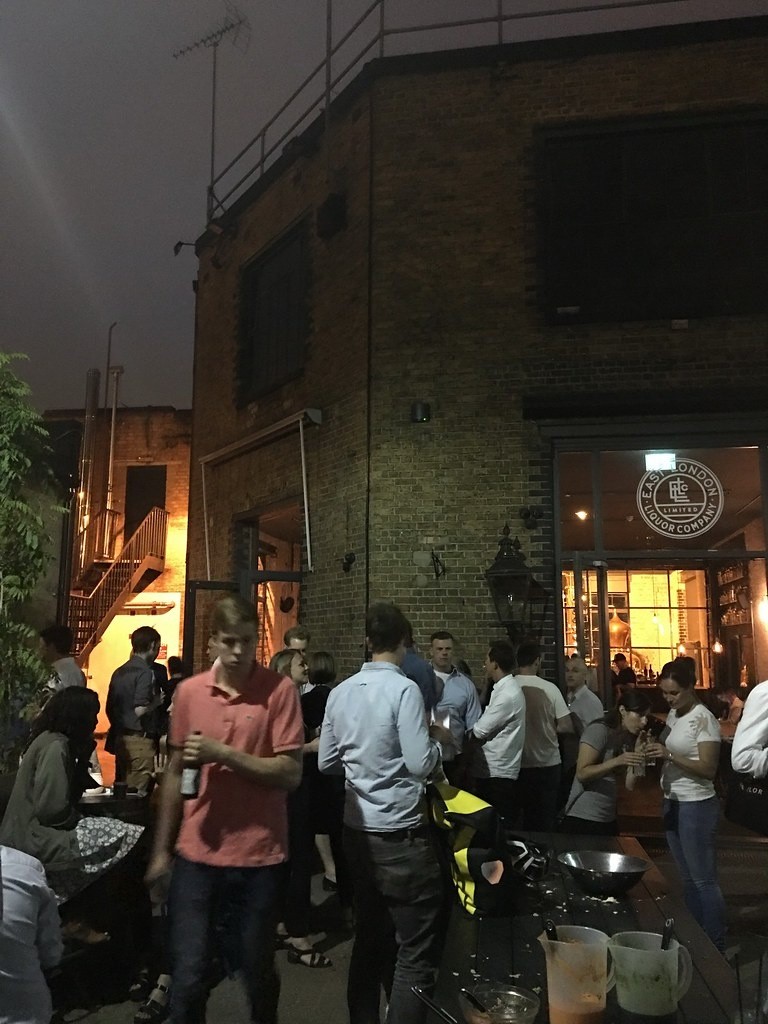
633;734;656;776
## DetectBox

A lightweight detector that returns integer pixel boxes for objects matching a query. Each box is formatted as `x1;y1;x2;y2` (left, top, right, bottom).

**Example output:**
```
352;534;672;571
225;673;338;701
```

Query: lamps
174;241;197;255
712;632;723;655
482;524;537;642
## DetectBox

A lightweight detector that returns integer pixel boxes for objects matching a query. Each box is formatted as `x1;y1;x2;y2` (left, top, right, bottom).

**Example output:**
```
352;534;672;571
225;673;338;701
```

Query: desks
421;832;768;1024
653;712;736;742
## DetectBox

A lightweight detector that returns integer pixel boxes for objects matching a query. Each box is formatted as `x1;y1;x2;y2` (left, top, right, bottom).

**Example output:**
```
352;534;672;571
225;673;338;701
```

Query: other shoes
322;875;338;891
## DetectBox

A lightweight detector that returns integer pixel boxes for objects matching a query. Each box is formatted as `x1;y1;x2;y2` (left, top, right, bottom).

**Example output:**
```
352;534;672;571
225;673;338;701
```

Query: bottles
180;730;202;800
716;562;748;625
644;664;653;679
114;777;128;799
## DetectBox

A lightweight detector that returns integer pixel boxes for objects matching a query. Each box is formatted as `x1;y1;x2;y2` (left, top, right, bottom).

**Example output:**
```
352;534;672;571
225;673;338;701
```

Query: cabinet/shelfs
716;576;751;627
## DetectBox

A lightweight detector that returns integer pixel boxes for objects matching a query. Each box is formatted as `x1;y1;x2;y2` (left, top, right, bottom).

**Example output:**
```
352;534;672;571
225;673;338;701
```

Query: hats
612;653;626;662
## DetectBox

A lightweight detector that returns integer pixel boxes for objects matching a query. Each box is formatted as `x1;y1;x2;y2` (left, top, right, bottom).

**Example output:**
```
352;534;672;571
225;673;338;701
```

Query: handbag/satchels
724;776;766;836
550;804;567;830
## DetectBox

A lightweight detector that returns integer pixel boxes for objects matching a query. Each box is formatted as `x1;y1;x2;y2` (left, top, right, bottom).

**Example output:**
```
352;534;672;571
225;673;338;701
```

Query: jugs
606;932;693;1017
537;925;616;1024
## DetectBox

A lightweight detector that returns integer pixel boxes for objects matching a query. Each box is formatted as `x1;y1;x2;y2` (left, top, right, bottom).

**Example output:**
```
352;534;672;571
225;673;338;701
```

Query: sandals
290;942;333;968
132;980;170;1023
127;960;153;1002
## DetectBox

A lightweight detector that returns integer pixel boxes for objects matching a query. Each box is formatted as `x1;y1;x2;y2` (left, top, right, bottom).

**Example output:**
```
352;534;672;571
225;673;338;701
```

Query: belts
122;729;158;741
366;828;429;843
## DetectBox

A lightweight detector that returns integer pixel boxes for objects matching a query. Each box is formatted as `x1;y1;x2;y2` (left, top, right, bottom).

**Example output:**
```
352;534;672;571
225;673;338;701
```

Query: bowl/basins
556;850;655;897
458;984;540;1024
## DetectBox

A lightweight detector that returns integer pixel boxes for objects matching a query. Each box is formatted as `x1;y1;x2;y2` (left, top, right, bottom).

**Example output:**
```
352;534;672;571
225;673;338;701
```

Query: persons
399;630;440;723
282;625;317;697
730;678;768;809
301;651;338;893
564;657;728;962
168;655;183;688
317;604;454;1024
512;641;573;833
32;625;87;717
270;648;331;968
427;631;482;835
104;626;182;793
610;653;637;683
142;593;307;1024
463;643;526;832
0;843;66;1024
564;657;604;729
712;680;756;725
0;686;149;1002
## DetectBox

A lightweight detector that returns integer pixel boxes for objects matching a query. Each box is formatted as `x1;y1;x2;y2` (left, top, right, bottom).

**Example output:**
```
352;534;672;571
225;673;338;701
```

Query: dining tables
77;789;149;826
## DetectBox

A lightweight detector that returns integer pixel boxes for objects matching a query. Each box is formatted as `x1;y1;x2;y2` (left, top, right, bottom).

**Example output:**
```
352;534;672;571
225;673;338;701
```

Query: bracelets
665;750;674;762
